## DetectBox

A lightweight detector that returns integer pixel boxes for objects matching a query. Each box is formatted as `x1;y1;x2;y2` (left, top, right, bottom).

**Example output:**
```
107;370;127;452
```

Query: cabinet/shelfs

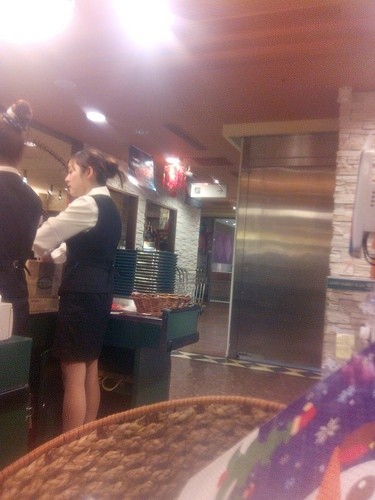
35;313;169;444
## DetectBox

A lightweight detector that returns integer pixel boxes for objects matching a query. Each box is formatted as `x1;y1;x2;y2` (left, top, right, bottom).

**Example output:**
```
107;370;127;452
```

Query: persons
0;99;43;335
33;149;127;435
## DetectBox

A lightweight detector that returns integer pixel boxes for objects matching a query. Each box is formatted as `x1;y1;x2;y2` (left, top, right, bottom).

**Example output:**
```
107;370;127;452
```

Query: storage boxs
24;260;61;314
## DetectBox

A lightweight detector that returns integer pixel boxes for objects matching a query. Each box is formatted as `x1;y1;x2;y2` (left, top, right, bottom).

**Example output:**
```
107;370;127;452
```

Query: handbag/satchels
24;259;64;314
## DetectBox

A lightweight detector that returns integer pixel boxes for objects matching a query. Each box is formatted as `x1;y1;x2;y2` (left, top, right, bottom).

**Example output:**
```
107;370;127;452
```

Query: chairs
0;398;293;500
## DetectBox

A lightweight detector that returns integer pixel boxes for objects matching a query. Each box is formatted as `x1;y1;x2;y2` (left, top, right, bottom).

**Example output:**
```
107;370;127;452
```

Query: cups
335;328;355;359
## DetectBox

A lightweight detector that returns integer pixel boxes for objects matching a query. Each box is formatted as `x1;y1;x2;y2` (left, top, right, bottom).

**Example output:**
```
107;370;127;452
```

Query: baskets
130;290;192;316
